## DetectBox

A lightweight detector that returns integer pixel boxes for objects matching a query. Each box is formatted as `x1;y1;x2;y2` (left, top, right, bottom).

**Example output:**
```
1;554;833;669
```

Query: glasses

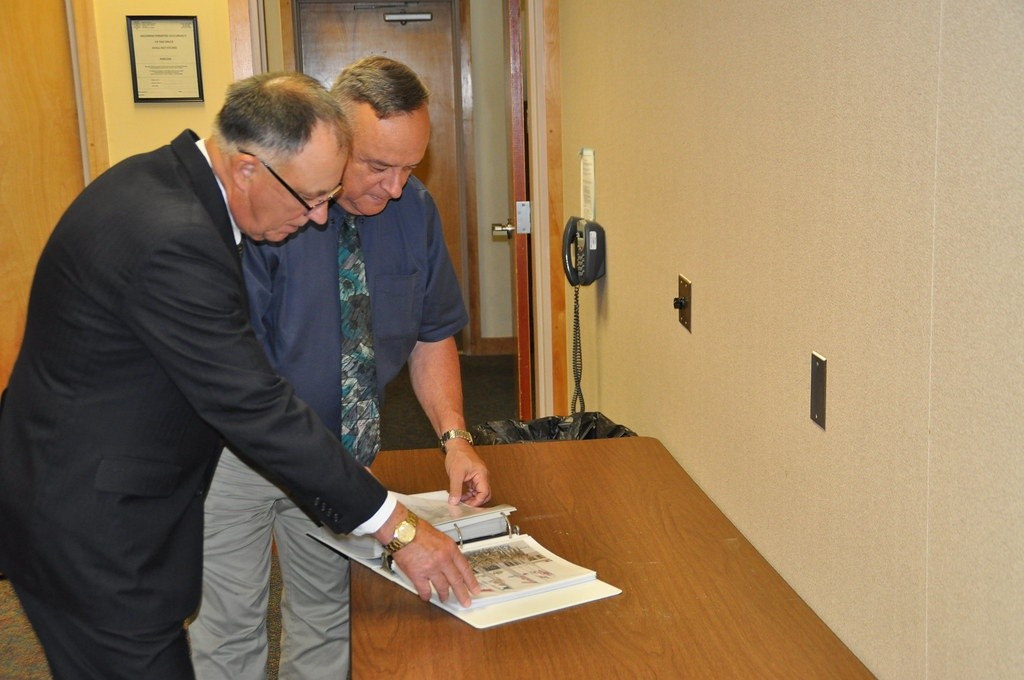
239;150;342;217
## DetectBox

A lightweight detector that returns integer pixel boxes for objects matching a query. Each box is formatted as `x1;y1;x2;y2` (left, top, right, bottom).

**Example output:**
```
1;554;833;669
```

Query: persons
0;72;483;679
187;56;494;680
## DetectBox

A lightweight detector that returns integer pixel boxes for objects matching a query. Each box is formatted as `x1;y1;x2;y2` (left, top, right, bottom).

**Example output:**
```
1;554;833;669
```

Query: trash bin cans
468;410;639;446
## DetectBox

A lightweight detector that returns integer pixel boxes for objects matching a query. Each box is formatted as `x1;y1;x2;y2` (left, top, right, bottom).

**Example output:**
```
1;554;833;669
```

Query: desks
349;437;879;680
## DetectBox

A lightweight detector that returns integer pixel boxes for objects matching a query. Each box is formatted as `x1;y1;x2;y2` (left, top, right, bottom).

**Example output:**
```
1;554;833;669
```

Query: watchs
379;509;421;555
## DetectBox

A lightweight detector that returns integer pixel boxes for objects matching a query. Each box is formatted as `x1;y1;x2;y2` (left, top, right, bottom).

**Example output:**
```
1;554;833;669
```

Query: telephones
562;215;606;288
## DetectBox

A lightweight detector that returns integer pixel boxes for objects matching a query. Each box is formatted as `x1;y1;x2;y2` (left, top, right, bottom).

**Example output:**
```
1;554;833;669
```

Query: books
305;488;624;632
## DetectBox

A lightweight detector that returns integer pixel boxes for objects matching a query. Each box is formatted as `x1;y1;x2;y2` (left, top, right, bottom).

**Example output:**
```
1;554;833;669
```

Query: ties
339;212;382;468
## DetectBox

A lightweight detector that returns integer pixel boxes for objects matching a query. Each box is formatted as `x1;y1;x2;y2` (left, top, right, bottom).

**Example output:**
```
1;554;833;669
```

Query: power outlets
678;274;692;334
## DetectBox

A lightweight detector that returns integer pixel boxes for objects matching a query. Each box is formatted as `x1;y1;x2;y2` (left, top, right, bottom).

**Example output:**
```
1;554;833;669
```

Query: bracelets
437;430;475;450
